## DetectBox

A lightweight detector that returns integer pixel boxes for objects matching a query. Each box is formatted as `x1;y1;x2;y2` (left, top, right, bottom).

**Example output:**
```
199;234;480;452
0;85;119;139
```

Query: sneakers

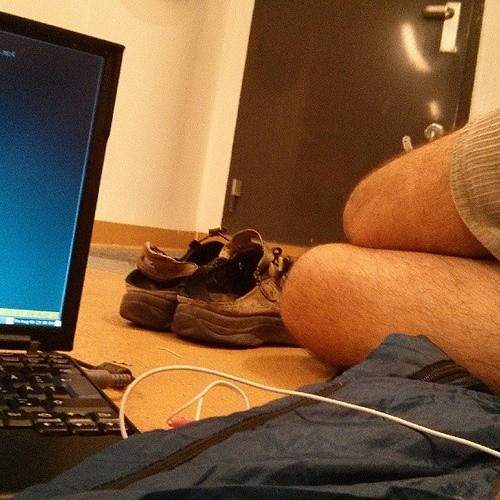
172;229;306;347
119;229;233;329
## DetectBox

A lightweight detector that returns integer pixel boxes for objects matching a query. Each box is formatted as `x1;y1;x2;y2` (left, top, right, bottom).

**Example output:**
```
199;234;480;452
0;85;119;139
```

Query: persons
280;107;500;395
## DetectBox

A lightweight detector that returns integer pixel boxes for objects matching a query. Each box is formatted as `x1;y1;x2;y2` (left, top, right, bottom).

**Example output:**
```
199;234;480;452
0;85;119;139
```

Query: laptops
0;12;142;493
